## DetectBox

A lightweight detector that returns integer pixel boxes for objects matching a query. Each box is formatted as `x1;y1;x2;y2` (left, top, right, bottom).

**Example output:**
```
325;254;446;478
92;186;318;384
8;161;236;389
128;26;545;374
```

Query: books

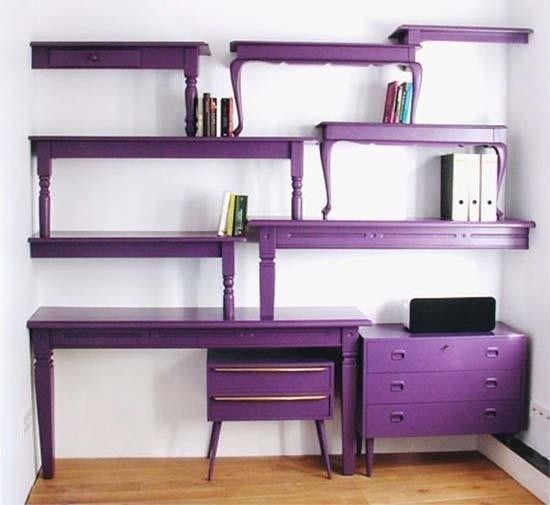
196;93;233;137
382;80;413;123
218;191;250;238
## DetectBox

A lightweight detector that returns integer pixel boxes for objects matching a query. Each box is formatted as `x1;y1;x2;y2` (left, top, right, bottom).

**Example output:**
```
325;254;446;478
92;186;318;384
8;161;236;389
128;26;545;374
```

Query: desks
27;305;372;481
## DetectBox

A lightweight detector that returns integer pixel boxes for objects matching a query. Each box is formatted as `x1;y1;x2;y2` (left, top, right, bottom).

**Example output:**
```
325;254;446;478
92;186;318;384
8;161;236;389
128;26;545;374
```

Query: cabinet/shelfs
358;321;530;477
202;349;337;480
27;23;537;322
